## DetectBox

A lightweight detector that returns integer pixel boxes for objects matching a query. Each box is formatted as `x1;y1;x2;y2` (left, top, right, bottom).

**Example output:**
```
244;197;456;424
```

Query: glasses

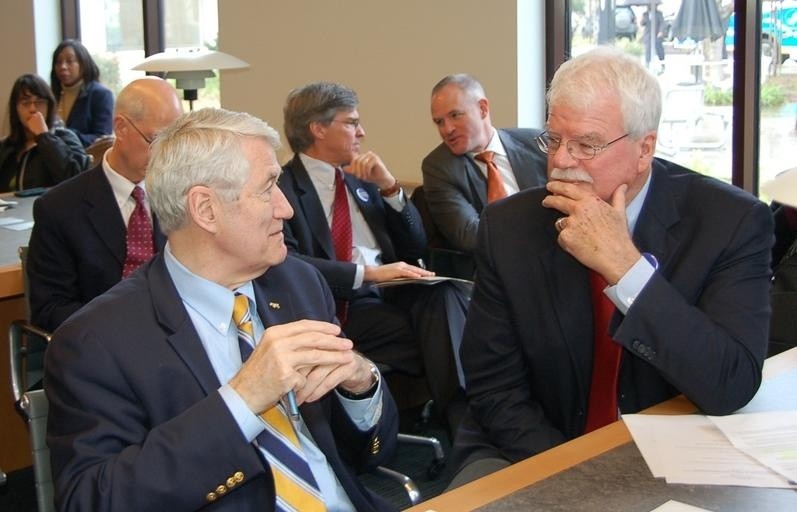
535;129;631;160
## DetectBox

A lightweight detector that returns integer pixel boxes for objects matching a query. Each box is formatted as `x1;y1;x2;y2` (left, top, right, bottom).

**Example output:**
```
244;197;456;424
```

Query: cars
581;4;636;43
662;10;676;42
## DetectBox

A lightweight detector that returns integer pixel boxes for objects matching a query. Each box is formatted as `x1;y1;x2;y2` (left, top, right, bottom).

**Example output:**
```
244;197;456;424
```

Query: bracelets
378;180;400;197
367;360;379;389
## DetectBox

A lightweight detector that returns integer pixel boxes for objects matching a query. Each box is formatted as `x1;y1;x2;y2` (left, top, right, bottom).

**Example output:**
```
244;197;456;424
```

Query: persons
640;4;668;75
442;47;780;497
0;73;92;195
21;74;184;391
45;38;114;145
47;108;404;512
273;80;514;484
419;71;553;256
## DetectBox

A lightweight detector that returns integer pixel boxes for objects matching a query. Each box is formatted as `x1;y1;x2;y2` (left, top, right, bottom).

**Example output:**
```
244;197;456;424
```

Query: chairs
5;245;53;430
18;387;426;511
84;137;112;167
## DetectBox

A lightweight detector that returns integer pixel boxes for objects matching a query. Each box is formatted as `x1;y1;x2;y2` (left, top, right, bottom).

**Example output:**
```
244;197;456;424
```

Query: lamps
133;43;251;113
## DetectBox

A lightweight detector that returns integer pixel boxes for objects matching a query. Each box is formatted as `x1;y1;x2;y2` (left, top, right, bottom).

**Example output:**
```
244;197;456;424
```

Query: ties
474;151;507;204
585;269;622;435
232;294;328;511
123;186;154;283
331;167;352;328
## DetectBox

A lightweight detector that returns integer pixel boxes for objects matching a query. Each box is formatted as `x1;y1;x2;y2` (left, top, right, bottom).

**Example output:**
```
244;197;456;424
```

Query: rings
556;216;564;229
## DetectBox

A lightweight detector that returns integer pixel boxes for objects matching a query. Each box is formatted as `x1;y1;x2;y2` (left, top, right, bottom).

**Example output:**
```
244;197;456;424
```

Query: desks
0;185;65;474
344;343;796;512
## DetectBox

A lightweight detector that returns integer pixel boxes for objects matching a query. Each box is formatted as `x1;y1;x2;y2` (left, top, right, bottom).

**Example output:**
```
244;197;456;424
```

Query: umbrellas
614;0;661;9
668;0;724;85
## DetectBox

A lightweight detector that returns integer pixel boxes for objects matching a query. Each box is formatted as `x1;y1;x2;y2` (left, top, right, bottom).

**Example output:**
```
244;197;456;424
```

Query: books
368;273;477;293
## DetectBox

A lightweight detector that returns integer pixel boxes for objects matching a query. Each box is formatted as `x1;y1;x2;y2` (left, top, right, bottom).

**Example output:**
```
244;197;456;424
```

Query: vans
725;0;797;63
